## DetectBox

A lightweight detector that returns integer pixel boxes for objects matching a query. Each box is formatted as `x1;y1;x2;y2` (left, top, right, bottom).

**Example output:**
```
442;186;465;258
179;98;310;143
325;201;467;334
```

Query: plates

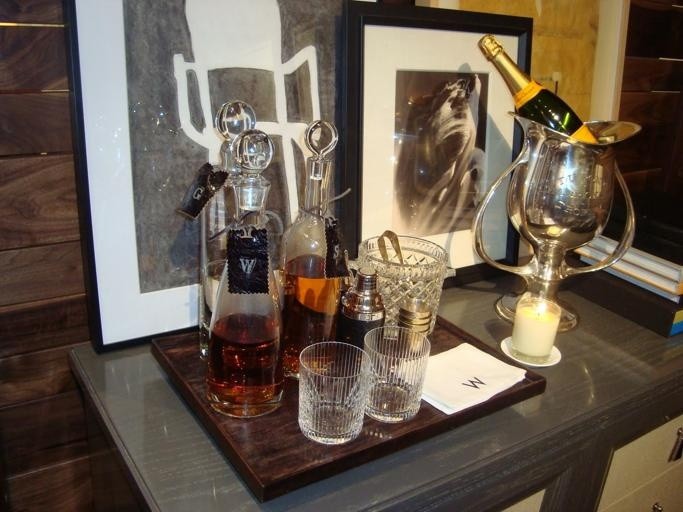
497;334;573;368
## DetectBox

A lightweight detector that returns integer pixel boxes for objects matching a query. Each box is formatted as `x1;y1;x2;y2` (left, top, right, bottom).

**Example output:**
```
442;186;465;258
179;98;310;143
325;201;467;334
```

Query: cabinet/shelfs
592;396;683;512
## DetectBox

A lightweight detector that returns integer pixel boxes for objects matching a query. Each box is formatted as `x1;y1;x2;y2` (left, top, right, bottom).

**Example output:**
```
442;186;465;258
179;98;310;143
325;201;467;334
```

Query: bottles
338;266;385;340
207;129;287;419
478;37;598;144
284;120;349;377
198;101;261;355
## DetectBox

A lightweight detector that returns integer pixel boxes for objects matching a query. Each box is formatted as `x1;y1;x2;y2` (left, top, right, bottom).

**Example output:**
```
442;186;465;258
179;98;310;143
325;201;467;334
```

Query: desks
52;249;682;510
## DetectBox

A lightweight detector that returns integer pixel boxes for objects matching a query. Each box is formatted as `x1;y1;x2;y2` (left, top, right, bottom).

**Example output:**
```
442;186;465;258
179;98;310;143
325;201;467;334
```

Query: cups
355;234;454;338
363;325;431;426
293;340;370;447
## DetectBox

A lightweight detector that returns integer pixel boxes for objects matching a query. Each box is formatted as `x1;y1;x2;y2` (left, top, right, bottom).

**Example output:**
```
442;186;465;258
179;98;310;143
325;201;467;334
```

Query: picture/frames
337;0;538;304
57;1;431;354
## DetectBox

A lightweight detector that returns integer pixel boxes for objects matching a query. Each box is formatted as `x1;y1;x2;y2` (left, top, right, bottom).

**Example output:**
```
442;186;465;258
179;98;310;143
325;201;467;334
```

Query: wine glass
476;113;661;336
